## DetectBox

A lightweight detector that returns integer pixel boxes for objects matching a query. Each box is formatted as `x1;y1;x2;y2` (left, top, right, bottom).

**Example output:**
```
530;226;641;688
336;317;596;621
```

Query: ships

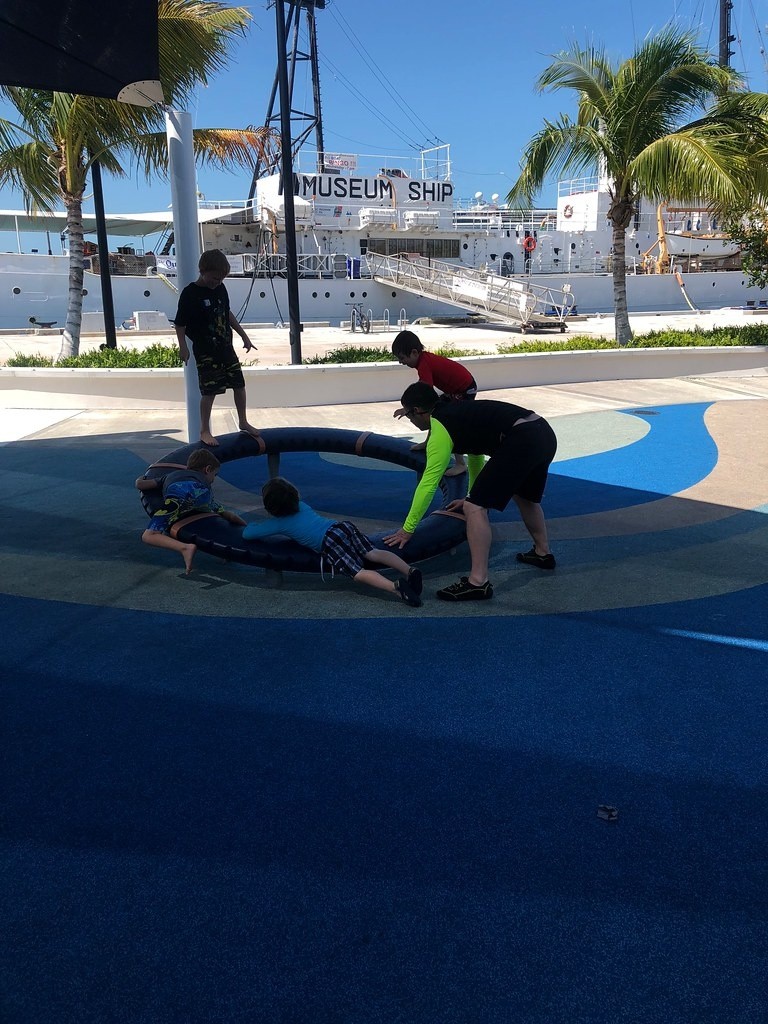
0;143;768;335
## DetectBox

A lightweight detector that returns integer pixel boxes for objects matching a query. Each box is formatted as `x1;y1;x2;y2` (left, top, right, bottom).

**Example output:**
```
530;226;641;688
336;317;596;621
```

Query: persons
134;450;247;576
172;249;263;447
242;476;423;609
382;382;557;600
394;330;479;476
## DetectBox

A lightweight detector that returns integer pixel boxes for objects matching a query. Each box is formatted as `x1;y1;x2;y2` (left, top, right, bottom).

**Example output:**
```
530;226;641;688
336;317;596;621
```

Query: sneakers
516;544;556;570
437;575;492;600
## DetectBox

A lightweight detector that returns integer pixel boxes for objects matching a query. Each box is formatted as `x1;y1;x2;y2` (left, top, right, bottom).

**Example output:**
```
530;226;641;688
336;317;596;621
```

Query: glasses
405;409;413;418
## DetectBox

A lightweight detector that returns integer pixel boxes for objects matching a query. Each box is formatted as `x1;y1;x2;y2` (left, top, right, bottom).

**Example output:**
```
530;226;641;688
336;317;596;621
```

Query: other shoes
407;567;423;596
394;580;421;608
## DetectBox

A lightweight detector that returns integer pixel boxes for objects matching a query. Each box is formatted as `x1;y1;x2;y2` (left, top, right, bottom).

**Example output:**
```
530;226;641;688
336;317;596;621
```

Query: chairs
731;300;768;310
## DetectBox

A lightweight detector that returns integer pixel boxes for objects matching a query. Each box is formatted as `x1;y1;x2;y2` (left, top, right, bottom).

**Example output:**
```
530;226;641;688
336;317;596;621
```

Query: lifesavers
524;237;536;251
